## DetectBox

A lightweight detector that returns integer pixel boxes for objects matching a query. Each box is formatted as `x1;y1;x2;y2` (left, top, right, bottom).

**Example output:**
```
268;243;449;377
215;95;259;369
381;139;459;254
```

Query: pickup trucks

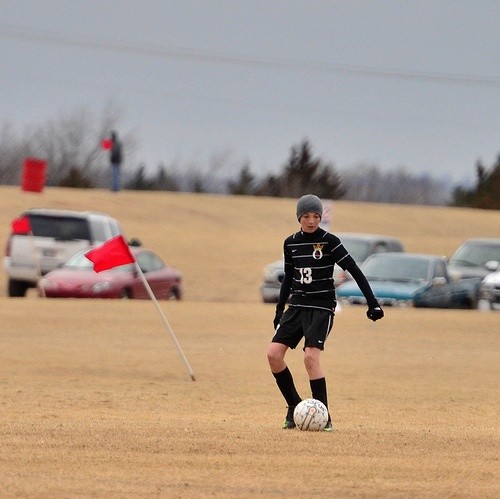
336;250;481;312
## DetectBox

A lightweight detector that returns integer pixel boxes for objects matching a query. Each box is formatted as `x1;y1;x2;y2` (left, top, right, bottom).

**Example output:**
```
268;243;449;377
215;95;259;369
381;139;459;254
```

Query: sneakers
281;405;297;429
323;413;333;431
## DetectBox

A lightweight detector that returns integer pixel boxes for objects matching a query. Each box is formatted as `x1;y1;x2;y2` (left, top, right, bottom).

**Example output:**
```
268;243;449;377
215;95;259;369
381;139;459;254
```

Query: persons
266;194;385;431
109;132;122;191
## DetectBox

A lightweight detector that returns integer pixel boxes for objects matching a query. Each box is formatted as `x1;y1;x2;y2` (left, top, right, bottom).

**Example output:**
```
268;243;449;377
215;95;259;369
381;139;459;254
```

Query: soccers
293;399;328;431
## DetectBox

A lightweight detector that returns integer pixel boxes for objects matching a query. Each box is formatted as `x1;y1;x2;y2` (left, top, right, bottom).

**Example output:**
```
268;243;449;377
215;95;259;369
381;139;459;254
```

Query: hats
296;194;323;220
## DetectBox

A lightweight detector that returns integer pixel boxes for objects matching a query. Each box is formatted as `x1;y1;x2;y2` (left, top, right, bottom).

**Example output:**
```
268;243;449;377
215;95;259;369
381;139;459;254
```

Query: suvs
5;208;141;298
260;232;402;301
447;238;500;310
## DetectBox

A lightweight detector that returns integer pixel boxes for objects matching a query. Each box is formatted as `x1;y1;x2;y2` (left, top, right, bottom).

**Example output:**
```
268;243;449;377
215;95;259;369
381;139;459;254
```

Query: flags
11;215;31;235
82;234;137;273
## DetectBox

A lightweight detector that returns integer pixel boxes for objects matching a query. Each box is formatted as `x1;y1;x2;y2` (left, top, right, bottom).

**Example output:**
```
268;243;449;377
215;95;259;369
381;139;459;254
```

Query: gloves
274;310;283;330
366;305;384;322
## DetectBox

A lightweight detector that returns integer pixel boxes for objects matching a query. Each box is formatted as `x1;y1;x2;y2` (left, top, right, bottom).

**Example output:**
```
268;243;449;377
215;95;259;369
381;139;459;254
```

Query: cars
38;242;184;301
